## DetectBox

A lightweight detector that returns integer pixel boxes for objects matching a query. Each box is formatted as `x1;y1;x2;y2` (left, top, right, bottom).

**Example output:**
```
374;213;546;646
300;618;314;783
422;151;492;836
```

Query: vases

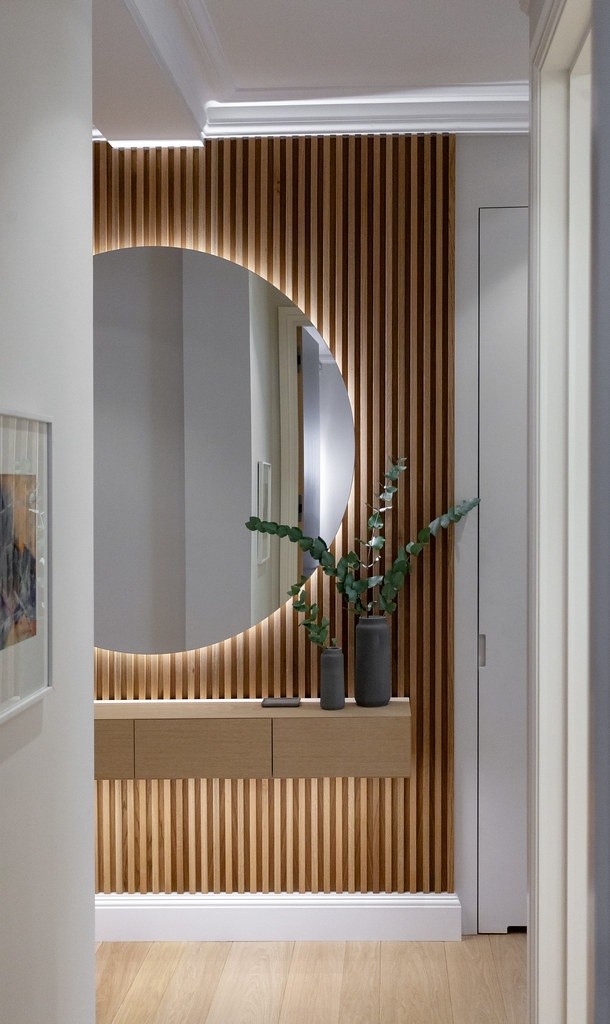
354;614;392;707
320;647;346;710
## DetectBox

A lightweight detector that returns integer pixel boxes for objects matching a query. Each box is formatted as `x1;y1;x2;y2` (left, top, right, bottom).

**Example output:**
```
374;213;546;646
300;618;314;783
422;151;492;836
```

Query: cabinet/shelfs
93;697;413;779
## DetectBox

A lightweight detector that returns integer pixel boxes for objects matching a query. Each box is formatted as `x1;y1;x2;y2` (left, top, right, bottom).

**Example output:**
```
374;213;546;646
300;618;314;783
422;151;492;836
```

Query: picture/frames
0;406;53;722
257;461;271;563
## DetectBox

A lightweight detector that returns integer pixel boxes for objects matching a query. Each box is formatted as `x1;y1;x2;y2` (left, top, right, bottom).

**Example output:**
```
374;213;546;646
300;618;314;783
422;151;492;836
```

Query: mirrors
94;247;355;657
